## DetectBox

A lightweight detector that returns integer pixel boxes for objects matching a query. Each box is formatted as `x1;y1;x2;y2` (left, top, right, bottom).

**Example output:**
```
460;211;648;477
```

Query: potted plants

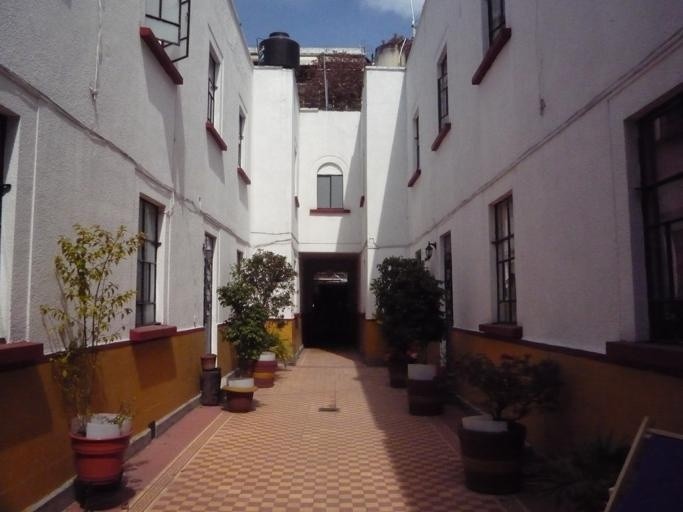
41;224;151;494
450;354;574;494
216;250;297;413
374;254;447;416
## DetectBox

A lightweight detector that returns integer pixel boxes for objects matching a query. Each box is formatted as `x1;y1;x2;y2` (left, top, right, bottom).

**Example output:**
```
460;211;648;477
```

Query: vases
201;353;216;371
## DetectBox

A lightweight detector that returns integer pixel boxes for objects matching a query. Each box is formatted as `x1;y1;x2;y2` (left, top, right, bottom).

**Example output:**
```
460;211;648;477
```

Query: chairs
603;414;683;512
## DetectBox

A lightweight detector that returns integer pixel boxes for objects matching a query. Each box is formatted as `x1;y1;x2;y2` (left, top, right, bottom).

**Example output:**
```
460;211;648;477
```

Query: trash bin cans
201;368;221;406
